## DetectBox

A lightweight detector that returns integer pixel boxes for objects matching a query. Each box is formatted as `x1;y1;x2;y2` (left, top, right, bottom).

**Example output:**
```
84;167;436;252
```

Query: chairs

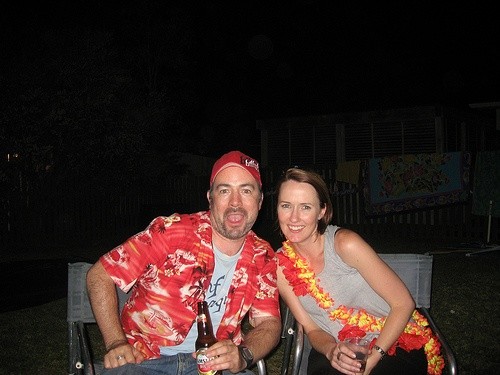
281;253;457;375
67;261;266;375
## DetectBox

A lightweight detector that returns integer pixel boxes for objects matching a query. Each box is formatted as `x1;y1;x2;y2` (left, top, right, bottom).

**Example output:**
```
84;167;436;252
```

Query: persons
273;168;444;375
86;152;283;375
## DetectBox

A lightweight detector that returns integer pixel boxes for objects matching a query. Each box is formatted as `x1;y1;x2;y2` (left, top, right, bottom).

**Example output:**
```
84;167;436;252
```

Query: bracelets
373;344;386;358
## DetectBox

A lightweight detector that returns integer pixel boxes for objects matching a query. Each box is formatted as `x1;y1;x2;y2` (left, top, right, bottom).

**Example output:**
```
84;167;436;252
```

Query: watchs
238;344;253;369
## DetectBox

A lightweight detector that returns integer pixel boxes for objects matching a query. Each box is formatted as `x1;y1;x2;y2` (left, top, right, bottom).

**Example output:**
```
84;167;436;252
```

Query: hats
210;151;262;189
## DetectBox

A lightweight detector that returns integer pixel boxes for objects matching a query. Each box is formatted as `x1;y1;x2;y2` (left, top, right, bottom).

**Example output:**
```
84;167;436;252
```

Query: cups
344;338;370;372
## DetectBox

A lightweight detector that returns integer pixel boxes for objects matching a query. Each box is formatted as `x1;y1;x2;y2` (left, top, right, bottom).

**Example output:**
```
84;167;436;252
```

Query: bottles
195;301;223;375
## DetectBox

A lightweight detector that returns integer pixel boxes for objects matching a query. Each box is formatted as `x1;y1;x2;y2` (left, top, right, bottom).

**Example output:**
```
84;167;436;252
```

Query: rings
336;352;344;360
117;355;124;361
